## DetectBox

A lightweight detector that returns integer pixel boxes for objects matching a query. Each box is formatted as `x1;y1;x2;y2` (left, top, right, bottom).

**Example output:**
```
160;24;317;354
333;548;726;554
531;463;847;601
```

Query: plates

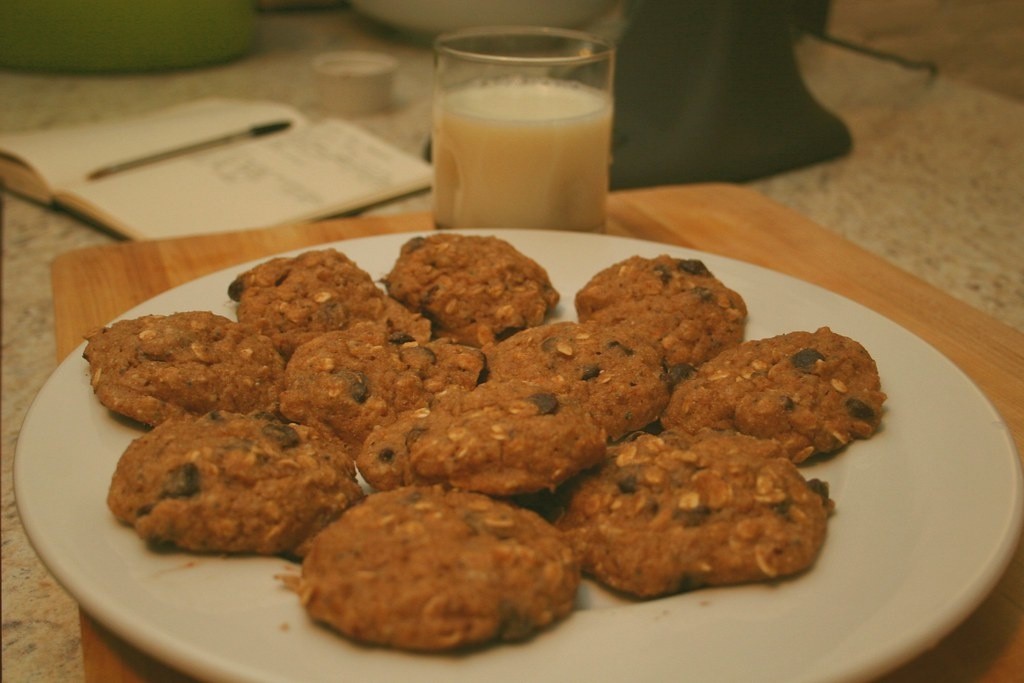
16;229;1022;683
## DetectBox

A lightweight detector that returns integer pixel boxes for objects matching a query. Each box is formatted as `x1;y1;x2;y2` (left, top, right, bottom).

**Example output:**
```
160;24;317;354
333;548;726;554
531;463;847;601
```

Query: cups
431;27;617;229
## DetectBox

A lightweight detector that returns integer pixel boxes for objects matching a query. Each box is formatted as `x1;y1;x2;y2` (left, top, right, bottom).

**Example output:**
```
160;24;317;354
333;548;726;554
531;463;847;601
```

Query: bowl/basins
316;51;399;115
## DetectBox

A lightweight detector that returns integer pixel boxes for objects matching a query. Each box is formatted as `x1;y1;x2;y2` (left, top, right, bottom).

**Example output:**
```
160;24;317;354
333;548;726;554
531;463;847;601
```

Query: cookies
82;234;887;649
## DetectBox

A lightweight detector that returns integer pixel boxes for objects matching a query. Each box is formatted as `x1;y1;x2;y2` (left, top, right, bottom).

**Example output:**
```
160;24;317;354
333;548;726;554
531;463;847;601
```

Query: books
0;97;433;240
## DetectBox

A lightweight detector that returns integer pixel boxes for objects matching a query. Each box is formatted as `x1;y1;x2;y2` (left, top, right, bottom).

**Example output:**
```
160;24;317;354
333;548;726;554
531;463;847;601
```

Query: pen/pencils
89;119;291;183
